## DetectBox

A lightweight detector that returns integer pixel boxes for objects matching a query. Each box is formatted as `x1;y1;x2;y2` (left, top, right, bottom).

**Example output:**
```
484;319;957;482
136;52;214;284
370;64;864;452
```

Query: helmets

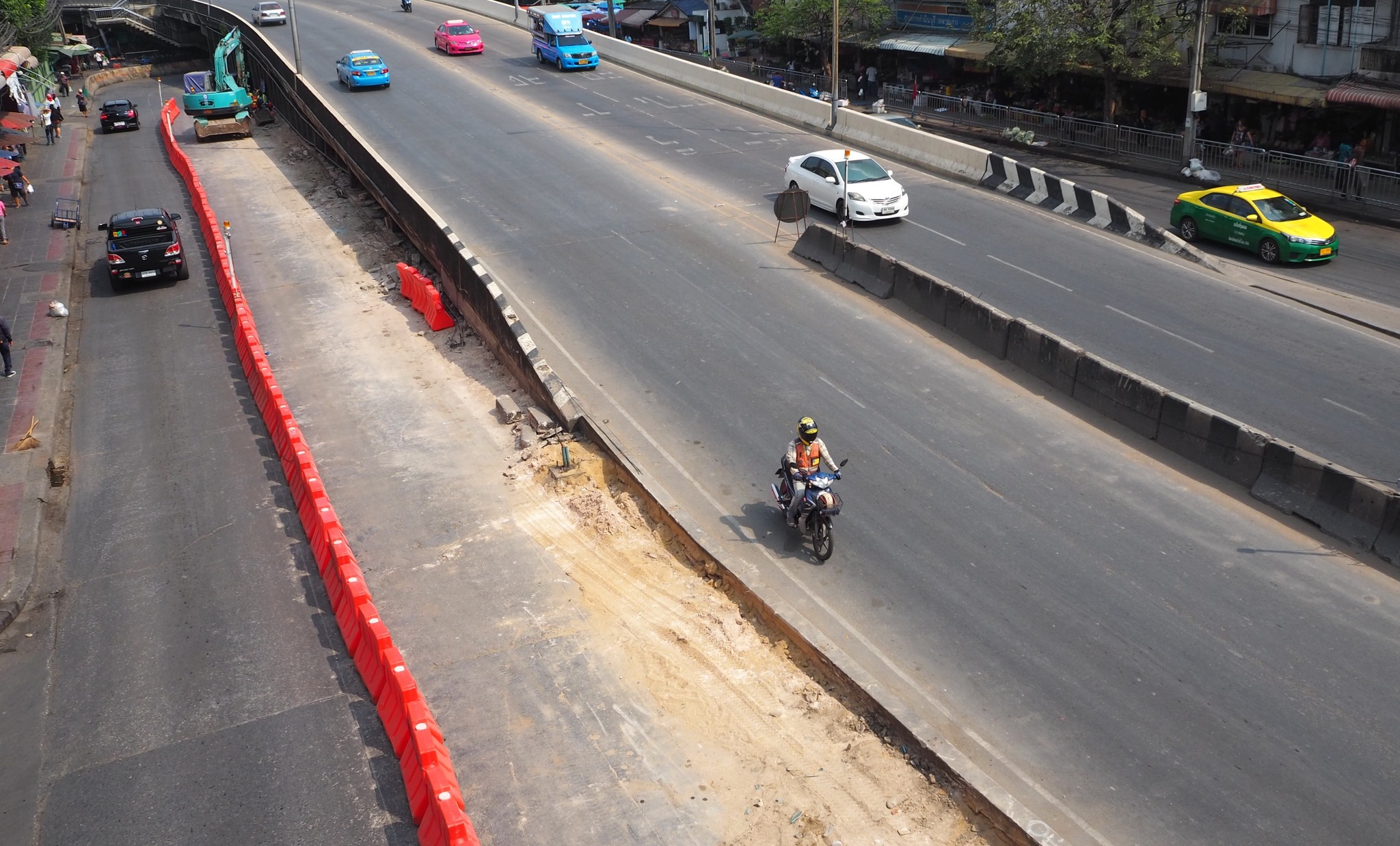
797;415;818;445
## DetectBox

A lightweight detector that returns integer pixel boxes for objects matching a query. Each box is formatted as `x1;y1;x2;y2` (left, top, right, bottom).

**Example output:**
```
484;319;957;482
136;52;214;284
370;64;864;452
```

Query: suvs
98;207;189;291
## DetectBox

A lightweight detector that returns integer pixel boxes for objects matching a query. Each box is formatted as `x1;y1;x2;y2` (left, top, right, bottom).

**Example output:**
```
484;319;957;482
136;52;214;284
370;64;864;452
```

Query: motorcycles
771;448;849;562
400;0;412;12
798;83;822;100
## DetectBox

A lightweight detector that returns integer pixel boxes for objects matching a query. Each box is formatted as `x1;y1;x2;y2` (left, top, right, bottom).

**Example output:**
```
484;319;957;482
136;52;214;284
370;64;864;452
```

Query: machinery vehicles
182;25;276;142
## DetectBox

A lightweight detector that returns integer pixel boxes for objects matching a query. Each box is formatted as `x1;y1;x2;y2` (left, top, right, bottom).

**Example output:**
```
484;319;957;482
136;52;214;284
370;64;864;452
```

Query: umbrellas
0;158;20;168
0;150;19;158
0;168;14;176
0;179;7;185
0;111;41;147
576;0;625;29
71;43;106;55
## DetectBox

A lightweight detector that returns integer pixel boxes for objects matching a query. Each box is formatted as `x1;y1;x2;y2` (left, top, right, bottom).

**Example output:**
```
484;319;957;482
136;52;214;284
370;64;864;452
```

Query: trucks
526;5;600;71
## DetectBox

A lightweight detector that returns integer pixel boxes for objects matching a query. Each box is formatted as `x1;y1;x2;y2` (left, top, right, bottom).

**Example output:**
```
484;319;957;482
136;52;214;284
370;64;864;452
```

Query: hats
79;88;83;93
60;71;64;75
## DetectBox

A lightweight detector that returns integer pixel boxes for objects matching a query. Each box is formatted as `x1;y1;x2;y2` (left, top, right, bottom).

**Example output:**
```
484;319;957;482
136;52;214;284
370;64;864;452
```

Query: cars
1170;183;1339;265
98;99;140;133
251;1;287;27
434;20;484;56
784;149;909;221
336;49;390;91
869;113;921;132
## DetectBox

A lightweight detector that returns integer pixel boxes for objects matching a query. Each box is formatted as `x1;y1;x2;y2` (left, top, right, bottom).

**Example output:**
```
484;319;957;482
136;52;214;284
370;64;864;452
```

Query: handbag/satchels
46;124;54;131
68;86;73;92
26;184;34;194
102;59;110;66
79;99;84;103
13;182;23;189
59;117;63;121
59;87;63;93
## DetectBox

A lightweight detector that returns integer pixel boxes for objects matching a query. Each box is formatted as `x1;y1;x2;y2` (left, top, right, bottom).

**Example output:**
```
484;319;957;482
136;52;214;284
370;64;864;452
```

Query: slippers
4;241;8;245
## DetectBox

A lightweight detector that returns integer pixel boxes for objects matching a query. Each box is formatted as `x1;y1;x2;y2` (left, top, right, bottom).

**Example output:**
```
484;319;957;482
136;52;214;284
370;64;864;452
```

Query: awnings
725;21;998;60
1207;0;1277;16
0;33;87;90
945;39;1400;109
603;7;690;28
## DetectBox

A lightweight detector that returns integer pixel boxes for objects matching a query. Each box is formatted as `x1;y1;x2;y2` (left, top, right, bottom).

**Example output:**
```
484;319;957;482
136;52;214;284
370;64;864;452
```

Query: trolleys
51;198;82;230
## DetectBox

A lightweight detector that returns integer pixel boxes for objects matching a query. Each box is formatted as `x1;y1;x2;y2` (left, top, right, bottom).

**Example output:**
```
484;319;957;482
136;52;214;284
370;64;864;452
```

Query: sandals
15;205;21;209
25;200;29;206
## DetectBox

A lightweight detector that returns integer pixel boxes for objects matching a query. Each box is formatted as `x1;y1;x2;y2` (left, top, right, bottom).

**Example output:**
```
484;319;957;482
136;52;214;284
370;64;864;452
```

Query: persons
0;315;16;377
402;0;406;10
786;417;842;527
3;146;30;208
751;57;832;88
83;50;110;71
625;34;632;43
0;200;8;244
1135;110;1366;203
461;27;470;34
857;63;1076;144
40;72;88;146
248;89;268;118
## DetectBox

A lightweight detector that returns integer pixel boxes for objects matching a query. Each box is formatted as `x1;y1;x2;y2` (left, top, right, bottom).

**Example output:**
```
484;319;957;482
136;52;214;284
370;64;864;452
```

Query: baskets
809;488;844;516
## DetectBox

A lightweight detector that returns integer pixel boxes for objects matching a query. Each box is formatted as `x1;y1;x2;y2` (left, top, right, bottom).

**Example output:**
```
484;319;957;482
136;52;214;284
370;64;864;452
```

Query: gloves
834;471;842;480
793;471;803;483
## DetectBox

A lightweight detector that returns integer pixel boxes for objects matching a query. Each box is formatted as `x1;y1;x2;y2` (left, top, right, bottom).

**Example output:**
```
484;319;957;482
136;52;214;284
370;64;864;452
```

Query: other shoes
101;69;105;71
47;141;50;146
62;95;67;97
56;134;62;139
52;142;56;146
6;370;15;378
785;512;796;527
